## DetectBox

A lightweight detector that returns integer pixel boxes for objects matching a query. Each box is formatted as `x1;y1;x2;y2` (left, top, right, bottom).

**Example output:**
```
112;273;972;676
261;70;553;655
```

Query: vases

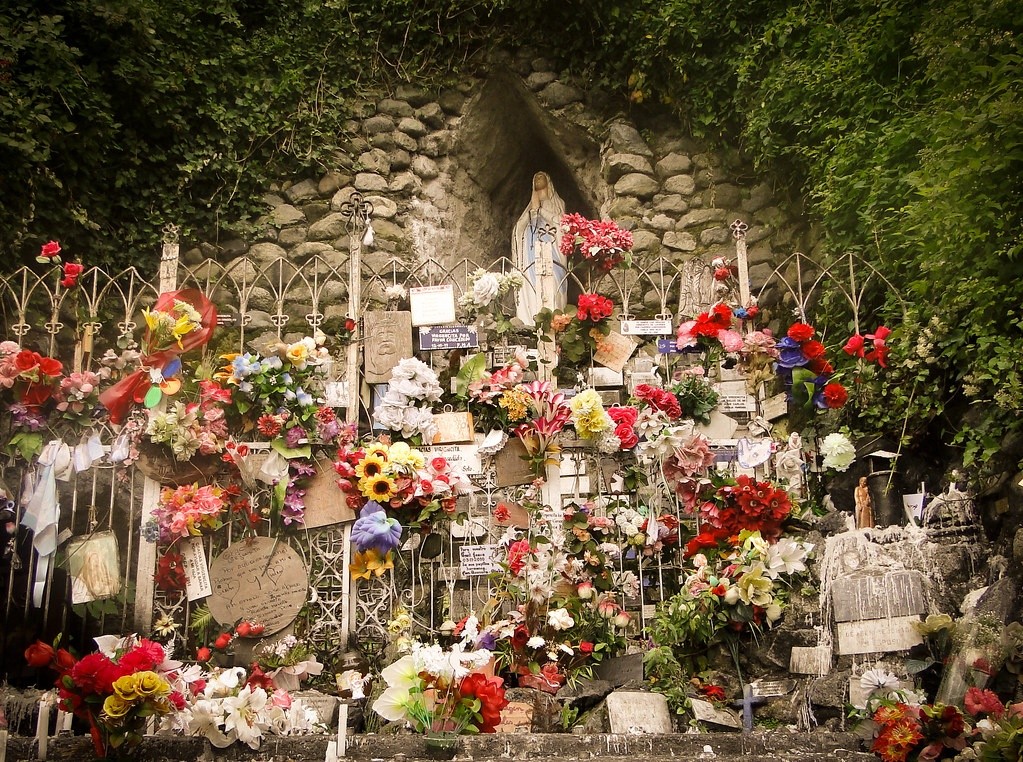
421;734;460;761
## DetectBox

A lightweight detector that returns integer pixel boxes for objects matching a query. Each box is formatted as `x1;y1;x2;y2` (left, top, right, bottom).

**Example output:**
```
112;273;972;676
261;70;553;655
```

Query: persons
854;477;874;529
510;172;569;379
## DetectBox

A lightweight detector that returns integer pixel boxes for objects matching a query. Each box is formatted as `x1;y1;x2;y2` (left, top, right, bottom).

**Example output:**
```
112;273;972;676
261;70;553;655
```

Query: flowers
0;213;1022;762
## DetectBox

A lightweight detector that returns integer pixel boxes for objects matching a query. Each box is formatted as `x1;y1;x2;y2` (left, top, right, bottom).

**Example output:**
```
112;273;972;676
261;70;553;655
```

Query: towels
22;441;73;556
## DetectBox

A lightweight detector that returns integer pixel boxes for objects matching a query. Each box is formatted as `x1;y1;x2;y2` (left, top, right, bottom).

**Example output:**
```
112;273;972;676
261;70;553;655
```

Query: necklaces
528;206;541;249
